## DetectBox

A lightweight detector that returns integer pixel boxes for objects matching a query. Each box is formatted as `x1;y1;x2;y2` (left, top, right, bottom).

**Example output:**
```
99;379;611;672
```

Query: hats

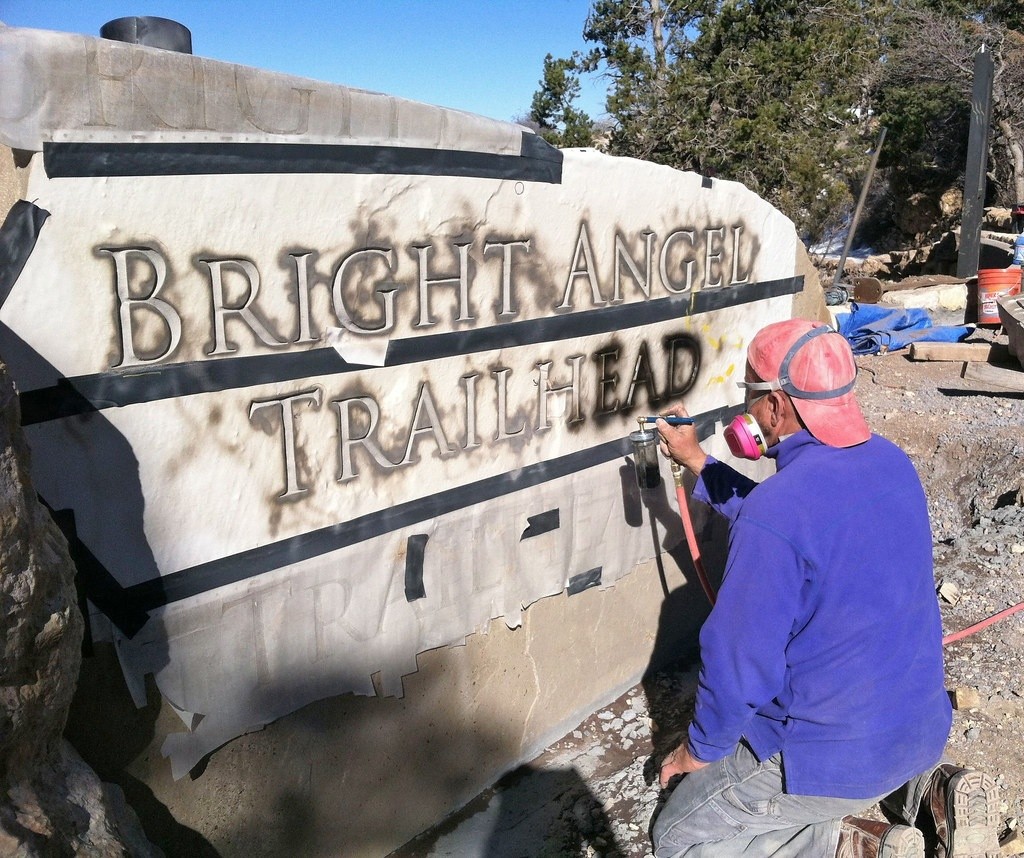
747;318;871;449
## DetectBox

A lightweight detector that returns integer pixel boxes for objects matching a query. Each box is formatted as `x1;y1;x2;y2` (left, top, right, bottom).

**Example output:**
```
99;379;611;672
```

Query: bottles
1013;236;1024;264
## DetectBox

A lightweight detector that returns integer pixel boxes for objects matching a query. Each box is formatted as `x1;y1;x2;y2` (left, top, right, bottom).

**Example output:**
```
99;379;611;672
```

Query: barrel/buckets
977;269;1022;324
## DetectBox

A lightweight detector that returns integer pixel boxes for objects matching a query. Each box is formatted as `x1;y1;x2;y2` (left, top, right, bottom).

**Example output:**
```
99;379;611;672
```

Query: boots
925;764;1001;858
835;814;926;858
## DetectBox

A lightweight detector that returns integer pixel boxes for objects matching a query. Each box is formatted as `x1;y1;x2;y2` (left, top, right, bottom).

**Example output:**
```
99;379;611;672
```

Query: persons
650;321;1001;858
